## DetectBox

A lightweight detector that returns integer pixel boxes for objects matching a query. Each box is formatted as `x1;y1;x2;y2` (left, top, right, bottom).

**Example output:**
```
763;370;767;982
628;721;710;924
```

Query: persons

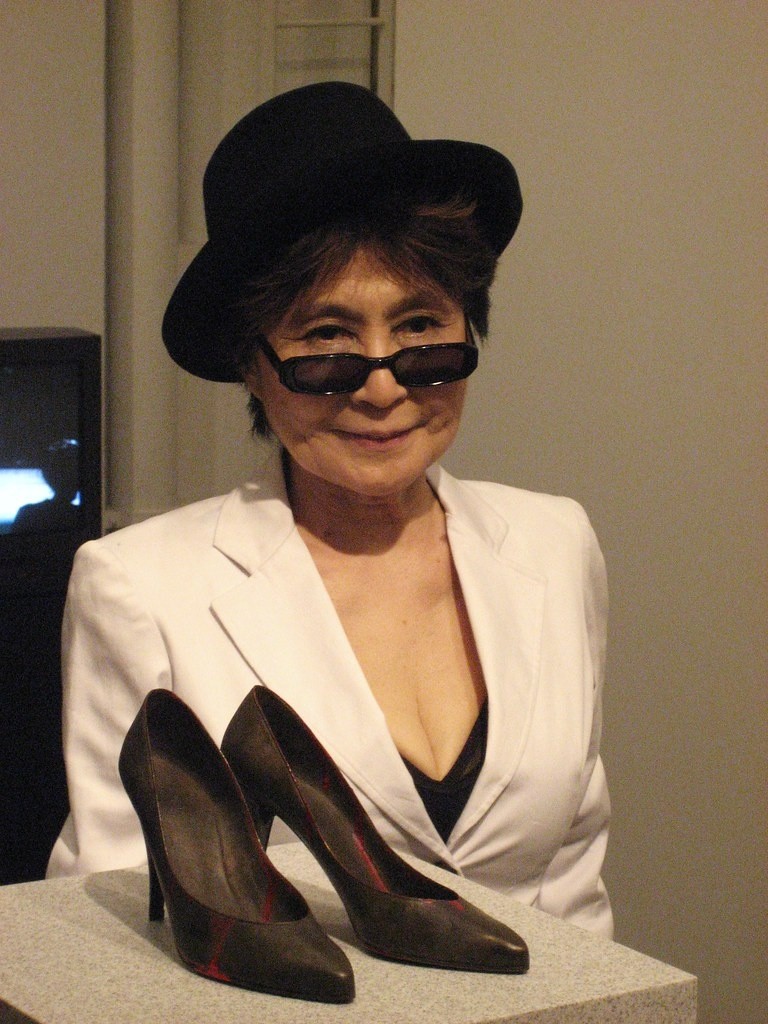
44;80;620;943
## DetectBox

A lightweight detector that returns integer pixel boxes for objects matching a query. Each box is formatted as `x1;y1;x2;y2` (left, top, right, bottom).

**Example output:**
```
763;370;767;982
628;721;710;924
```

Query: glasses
256;314;480;395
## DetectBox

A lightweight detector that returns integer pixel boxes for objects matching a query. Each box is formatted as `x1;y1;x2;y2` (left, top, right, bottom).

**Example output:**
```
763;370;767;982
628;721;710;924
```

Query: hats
160;80;523;383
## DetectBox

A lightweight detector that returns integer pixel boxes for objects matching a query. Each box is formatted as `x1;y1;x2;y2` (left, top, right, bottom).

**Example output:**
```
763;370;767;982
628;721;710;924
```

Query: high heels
118;688;358;1004
217;684;530;975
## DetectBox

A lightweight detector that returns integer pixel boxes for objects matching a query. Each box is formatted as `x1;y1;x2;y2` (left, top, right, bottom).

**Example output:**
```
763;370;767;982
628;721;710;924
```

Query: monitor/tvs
0;325;104;600
